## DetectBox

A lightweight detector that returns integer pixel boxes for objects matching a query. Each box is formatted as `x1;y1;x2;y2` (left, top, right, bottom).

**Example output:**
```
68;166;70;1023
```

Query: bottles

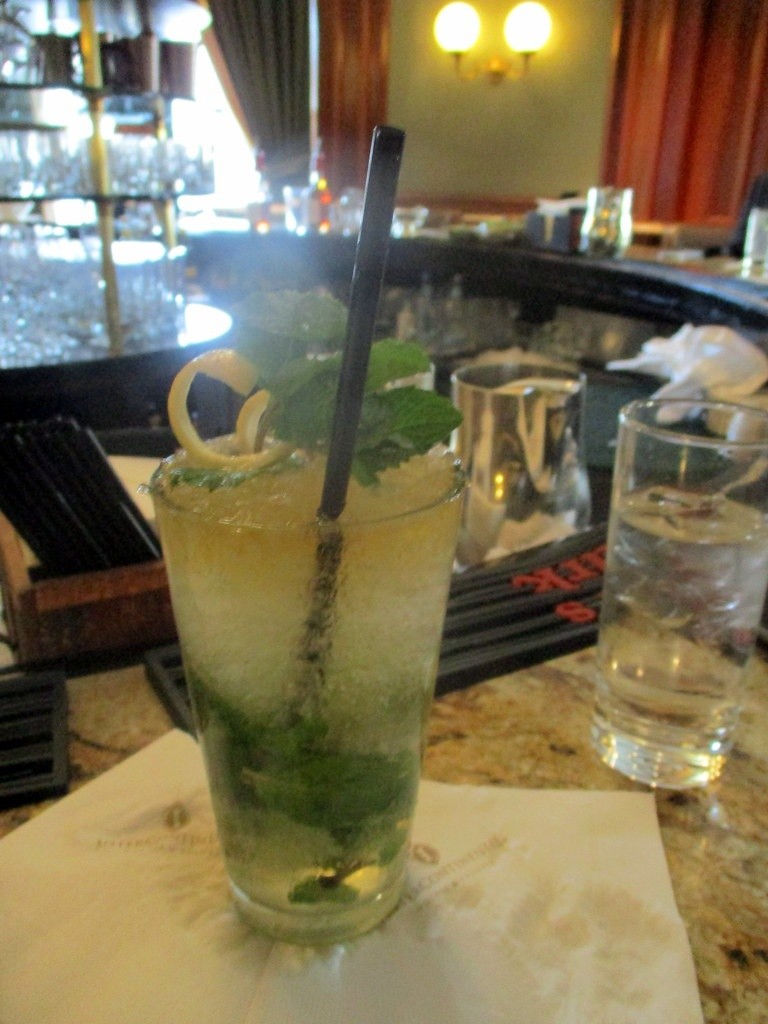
299;155;331;236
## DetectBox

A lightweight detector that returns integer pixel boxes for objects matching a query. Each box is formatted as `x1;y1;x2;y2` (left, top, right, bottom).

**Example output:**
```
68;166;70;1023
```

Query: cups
580;186;634;261
588;398;768;791
451;364;587;571
150;436;470;948
0;0;221;364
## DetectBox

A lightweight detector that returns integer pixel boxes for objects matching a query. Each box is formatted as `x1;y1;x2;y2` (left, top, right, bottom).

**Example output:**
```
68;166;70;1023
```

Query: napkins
0;726;707;1024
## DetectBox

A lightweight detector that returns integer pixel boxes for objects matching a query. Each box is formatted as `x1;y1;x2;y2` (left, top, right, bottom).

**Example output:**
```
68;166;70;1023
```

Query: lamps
432;1;552;87
